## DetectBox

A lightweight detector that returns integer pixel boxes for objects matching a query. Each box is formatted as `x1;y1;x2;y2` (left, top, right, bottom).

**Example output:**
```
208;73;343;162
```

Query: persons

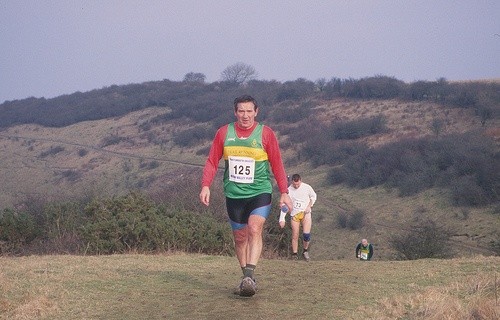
356;238;374;261
200;95;293;296
278;174;317;262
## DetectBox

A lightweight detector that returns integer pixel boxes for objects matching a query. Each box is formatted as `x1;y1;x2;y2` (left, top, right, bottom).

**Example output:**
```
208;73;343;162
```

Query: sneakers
302;252;310;260
234;277;256;297
291;255;298;262
